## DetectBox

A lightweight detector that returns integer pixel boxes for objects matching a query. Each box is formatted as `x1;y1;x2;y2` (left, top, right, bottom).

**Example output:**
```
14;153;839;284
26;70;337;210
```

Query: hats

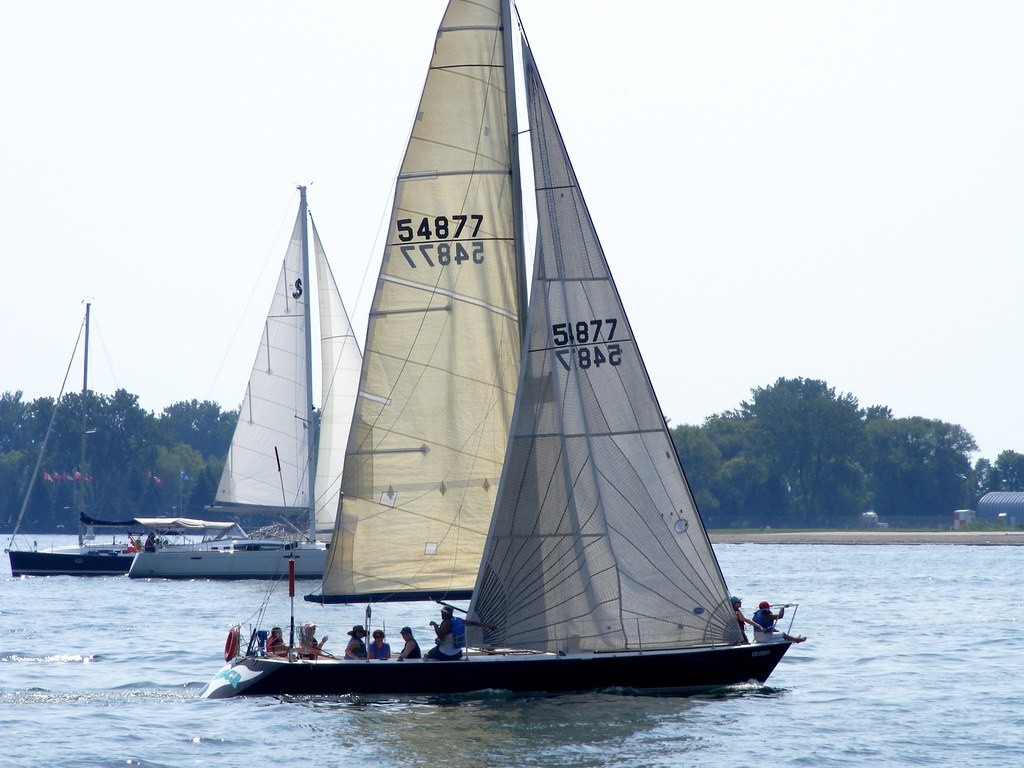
400;627;411;634
759;601;772;608
440;606;453;614
304;623;316;630
732;596;741;604
348;626;370;636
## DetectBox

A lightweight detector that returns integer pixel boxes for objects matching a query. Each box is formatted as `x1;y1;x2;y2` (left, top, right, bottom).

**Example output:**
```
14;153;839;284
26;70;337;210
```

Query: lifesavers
224;629;237;662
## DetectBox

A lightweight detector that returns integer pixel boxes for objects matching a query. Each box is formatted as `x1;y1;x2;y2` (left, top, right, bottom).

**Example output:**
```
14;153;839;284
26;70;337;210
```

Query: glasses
376;636;383;638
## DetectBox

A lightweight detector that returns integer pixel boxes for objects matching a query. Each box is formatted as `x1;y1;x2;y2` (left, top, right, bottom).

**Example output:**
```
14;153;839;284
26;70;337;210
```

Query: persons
302;623;337;660
752;602;807;644
424;606;467;662
266;626;290;660
344;625;391;660
397;627;421;659
728;597;765;645
144;531;156;553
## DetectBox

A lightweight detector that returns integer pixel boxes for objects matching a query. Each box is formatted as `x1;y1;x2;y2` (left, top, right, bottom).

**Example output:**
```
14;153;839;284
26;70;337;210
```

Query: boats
156;515;185;535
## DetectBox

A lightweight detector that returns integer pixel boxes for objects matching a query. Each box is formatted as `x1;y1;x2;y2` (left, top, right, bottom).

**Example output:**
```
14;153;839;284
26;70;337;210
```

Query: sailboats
4;302;234;577
195;0;798;698
125;186;362;578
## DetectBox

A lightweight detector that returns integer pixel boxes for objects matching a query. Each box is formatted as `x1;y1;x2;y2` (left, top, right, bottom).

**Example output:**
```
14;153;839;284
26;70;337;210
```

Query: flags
43;470;93;484
180;472;193;482
147;470;161;484
